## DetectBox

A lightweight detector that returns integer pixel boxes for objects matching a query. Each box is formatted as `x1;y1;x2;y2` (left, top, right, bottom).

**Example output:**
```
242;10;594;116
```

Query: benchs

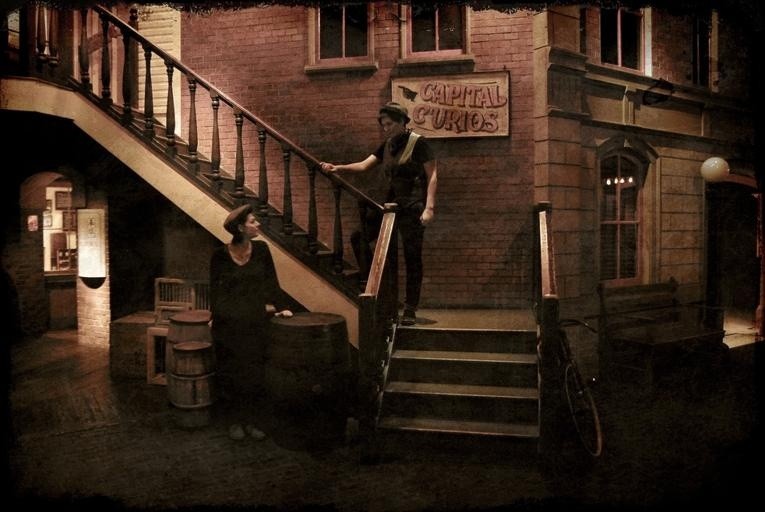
596;277;726;392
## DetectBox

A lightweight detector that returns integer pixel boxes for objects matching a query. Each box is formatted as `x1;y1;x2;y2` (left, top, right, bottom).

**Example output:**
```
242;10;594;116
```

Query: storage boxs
109;311;155;378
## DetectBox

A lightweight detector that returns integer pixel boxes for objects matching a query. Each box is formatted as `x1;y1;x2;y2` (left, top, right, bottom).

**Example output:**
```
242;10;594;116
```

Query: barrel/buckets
268;311;349;452
165;308;220;383
168;341;217;409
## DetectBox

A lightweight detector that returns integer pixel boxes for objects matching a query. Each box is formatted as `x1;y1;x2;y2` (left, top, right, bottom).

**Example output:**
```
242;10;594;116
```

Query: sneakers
401;311;416;324
246;425;266;441
228;425;246;441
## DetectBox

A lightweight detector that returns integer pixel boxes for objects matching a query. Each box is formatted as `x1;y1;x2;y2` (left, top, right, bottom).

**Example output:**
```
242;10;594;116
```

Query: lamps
701;157;729;182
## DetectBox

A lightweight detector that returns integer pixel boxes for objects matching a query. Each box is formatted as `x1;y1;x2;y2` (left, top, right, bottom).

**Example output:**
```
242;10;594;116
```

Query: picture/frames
43;192;87;231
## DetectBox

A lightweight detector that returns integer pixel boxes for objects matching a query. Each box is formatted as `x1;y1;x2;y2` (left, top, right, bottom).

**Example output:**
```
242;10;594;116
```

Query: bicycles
536;318;603;460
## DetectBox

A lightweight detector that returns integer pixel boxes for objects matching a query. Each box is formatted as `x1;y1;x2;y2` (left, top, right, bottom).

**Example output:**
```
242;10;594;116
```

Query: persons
207;205;293;442
320;104;437;327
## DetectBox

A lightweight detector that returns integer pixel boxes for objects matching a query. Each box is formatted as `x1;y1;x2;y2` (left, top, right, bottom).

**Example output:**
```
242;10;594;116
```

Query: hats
223;203;253;229
380;101;410;124
